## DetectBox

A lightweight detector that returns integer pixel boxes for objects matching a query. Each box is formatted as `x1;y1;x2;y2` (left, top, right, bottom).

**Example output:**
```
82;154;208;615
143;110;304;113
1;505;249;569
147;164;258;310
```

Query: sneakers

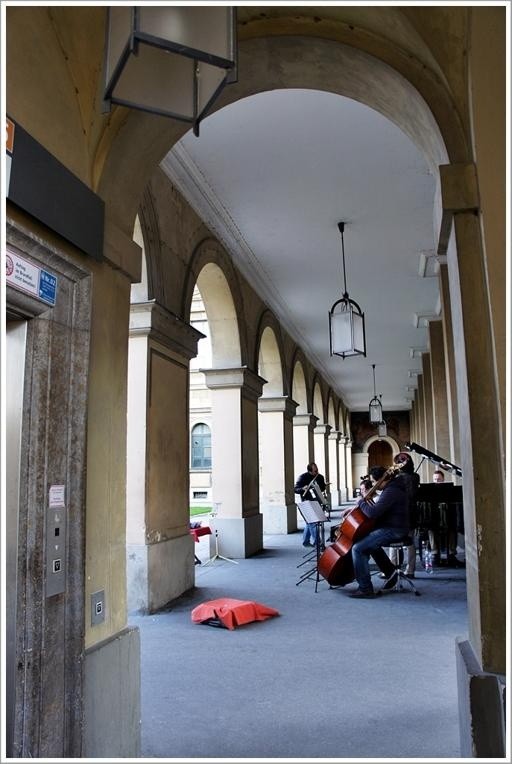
447;558;466;568
422;560;440;568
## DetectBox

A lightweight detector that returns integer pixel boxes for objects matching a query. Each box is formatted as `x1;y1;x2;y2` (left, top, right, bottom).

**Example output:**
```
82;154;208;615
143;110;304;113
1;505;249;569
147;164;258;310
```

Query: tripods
201;530;239;567
296;507;326;593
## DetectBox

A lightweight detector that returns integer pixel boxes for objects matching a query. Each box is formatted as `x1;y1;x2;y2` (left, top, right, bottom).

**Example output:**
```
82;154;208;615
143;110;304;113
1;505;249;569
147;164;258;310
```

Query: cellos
317;459;409;585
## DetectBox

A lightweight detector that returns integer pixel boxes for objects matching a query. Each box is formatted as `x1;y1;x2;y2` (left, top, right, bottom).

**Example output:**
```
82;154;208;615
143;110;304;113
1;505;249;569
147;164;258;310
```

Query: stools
373;536;422;597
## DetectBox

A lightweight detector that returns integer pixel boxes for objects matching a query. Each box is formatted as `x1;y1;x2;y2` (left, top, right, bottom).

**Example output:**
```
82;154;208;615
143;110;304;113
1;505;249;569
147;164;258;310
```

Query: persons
360;481;380;502
294;463;326;547
381;453;419;578
347;467;408;597
428;471;464;568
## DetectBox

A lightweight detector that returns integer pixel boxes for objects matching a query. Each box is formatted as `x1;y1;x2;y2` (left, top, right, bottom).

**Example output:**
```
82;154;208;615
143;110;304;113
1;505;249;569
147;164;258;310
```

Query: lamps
99;10;241;135
319;216;386;436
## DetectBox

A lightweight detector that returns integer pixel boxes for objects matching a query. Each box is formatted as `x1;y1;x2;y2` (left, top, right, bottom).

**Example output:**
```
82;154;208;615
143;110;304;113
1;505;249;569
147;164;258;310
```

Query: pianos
404;441;464;531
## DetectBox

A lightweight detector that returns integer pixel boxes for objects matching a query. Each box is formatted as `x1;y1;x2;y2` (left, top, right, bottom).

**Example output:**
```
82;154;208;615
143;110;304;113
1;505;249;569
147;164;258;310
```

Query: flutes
303;472;318;498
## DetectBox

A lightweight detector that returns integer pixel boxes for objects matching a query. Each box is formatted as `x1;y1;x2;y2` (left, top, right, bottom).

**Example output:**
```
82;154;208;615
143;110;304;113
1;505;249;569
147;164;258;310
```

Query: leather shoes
304;543;318;549
379;573;414;589
344;587;373;598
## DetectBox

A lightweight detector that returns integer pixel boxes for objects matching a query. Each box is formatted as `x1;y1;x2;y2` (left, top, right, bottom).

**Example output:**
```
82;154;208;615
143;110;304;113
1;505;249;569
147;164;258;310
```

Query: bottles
425;553;433;577
